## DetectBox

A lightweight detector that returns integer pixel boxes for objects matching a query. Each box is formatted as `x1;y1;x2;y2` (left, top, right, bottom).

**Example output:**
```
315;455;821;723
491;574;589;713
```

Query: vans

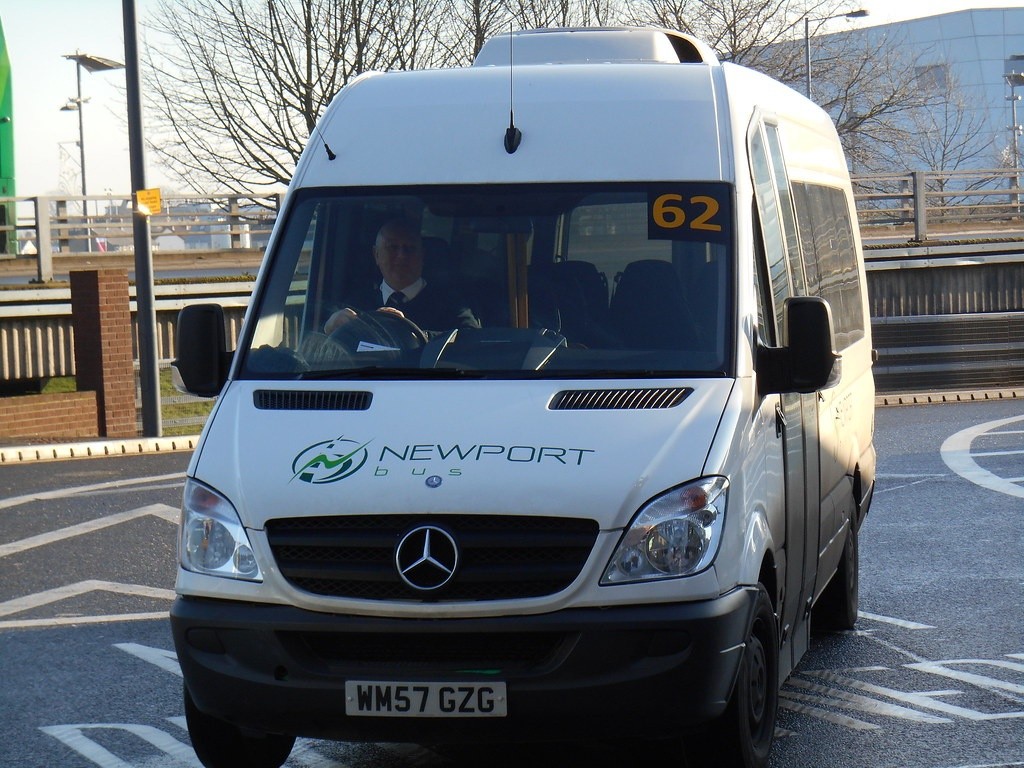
171;22;881;768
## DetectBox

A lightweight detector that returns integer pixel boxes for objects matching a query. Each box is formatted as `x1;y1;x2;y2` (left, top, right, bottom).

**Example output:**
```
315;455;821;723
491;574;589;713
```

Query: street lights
803;8;872;99
1002;71;1024;219
57;48;126;252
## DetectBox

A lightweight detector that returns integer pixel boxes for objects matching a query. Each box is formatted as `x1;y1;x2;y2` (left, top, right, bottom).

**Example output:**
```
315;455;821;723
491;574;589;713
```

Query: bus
0;16;17;257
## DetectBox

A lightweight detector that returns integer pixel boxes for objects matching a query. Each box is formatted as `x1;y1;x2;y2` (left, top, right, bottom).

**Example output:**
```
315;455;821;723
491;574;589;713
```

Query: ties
390;292;405;313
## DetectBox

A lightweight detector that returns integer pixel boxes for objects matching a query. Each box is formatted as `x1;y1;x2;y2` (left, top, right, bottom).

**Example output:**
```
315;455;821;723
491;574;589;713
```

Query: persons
323;220;480;343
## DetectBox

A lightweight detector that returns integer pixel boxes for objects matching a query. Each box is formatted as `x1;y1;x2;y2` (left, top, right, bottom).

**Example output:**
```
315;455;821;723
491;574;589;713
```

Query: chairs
419;237;721;349
